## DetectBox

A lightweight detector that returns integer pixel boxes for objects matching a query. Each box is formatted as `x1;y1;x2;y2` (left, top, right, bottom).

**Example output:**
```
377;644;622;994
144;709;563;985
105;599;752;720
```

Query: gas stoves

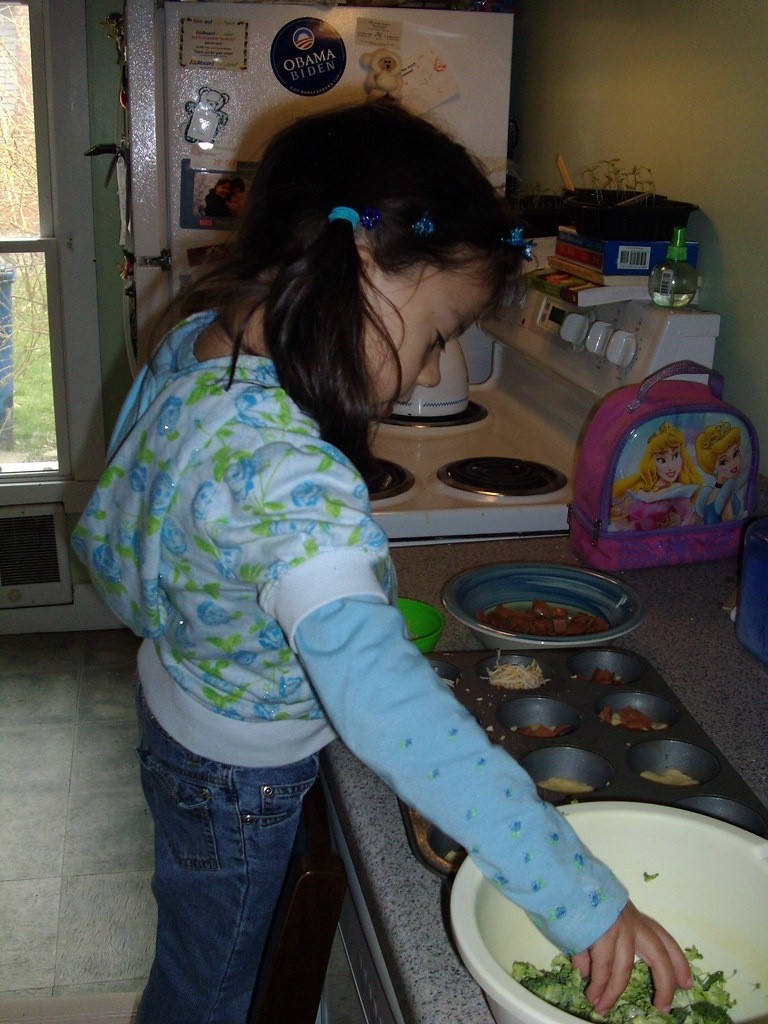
326;264;722;541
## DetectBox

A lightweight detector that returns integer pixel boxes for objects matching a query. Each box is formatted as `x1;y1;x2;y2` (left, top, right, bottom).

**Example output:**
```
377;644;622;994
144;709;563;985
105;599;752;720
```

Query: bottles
647;225;699;307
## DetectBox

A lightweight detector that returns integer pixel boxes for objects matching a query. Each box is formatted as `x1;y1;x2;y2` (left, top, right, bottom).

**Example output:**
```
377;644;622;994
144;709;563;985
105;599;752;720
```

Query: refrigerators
116;0;514;393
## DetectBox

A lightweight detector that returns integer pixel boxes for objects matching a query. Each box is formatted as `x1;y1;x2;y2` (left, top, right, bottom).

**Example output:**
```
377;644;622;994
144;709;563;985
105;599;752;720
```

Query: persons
205;177;248;217
73;95;691;1024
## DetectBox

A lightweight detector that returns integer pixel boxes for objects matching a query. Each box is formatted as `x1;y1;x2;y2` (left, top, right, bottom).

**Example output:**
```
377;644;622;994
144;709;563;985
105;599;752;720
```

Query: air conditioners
0;501;75;610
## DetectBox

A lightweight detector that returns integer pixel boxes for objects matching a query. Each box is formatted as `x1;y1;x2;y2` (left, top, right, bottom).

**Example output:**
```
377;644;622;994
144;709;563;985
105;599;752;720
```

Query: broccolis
512;932;736;1024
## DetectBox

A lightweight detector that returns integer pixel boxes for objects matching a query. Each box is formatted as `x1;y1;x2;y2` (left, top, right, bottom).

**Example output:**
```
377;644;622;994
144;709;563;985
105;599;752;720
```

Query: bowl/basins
441;562;647;647
450;803;768;1024
396;596;446;653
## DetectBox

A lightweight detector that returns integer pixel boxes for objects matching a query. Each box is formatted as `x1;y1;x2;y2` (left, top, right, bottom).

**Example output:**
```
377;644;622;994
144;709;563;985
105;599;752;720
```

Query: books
526;232;702;308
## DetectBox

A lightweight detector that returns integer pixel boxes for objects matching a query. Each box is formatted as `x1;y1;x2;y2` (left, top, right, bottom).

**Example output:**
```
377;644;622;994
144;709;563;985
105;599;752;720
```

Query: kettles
391;337;470;418
